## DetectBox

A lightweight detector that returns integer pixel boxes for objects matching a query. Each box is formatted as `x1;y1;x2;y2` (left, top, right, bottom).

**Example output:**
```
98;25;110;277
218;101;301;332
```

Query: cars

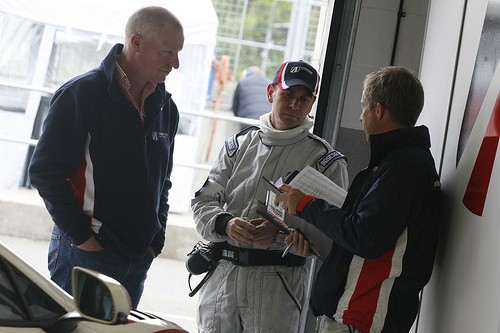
0;241;191;333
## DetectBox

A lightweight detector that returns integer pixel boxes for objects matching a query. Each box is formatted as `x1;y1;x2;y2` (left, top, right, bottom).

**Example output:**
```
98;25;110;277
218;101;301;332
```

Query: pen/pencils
282;242;293;258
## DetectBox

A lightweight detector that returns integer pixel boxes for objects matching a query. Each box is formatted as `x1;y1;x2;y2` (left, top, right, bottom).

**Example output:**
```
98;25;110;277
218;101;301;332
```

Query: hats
273;60;320;97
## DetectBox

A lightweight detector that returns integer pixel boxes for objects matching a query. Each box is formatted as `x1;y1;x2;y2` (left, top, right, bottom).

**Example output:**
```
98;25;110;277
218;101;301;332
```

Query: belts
217;243;306;267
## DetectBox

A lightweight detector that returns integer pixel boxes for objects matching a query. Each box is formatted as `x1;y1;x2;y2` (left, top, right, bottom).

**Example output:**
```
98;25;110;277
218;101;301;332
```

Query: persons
274;66;442;333
27;6;184;311
190;60;350;333
232;66;274;132
